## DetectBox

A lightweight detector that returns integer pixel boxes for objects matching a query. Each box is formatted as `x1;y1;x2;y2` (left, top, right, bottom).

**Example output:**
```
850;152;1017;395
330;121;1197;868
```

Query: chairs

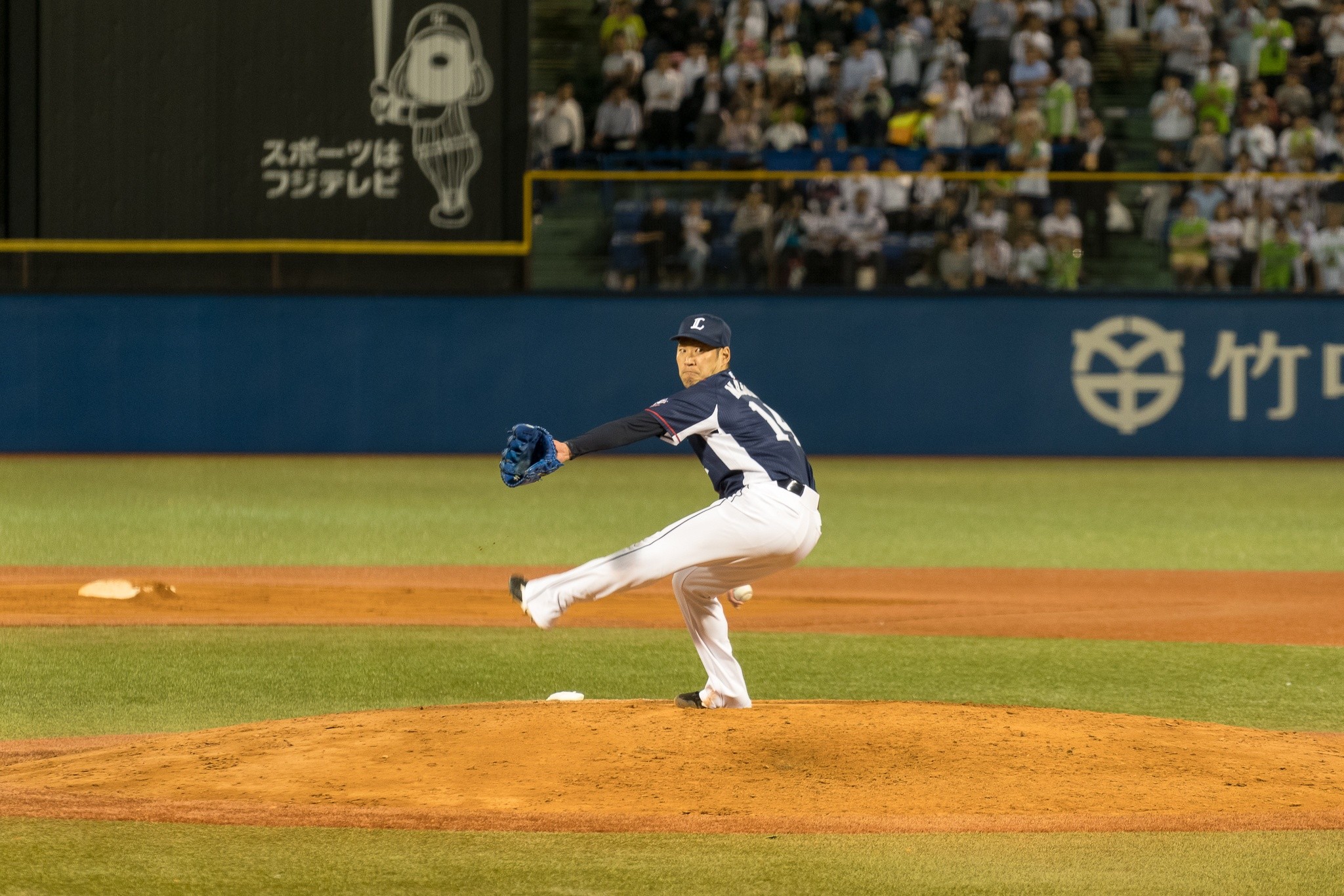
574;0;1344;295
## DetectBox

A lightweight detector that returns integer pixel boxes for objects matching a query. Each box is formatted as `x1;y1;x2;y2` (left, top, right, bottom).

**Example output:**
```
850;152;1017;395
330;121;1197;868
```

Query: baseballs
733;585;752;601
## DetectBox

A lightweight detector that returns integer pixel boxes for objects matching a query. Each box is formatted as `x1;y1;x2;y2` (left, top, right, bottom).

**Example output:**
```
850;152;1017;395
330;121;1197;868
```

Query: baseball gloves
499;423;565;487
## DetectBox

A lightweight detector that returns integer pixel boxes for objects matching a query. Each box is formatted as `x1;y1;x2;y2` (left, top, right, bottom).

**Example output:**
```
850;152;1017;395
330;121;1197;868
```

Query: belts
776;477;806;500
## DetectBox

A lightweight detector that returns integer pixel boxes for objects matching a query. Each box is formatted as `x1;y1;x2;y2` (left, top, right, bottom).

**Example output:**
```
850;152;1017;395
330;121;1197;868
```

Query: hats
1023;30;1055;59
669;313;732;349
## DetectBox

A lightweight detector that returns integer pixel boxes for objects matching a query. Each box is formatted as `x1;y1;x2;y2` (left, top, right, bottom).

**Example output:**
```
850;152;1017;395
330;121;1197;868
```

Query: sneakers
508;574;550;630
673;690;753;710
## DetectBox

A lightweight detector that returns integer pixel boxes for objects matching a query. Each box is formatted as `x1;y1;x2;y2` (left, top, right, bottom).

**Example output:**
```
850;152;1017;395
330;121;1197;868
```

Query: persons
525;0;1344;296
497;312;820;710
